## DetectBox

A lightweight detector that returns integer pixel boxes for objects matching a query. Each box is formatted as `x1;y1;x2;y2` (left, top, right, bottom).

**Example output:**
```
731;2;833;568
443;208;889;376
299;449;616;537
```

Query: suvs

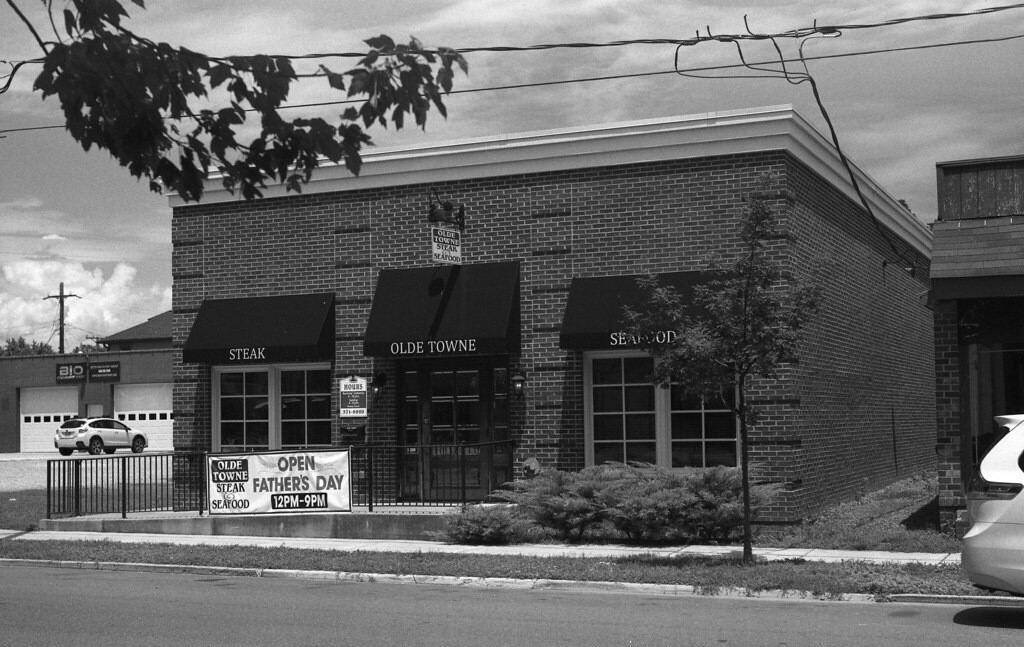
960;414;1024;596
53;416;149;456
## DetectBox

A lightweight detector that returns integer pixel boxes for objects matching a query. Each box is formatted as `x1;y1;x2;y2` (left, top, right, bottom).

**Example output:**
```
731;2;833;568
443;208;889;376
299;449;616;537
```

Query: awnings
363;260;520;358
559;269;734;349
182;293;336;363
